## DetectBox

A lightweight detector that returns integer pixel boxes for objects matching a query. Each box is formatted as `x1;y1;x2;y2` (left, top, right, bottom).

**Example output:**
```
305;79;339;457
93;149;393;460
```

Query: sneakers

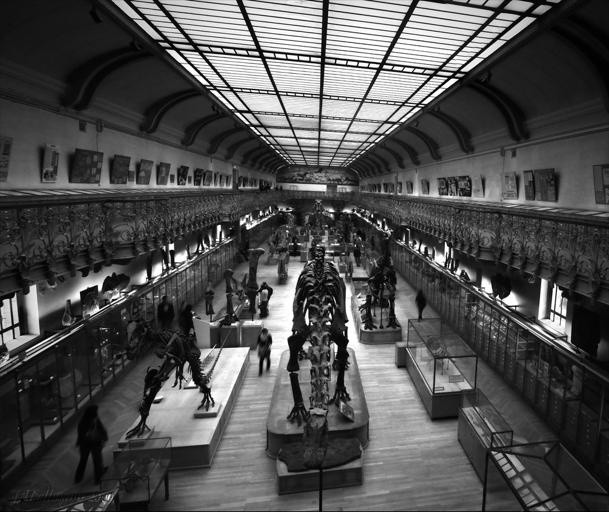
73;476;83;482
96;467;109;485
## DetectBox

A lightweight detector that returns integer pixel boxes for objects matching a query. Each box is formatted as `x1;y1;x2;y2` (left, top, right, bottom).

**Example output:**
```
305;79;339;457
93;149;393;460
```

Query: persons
257;282;274;313
369;235;376;250
253;327;273;377
73;401;110;484
157;294;174;330
258;284;269;318
415;289;426;324
352;243;361;266
177;303;199;349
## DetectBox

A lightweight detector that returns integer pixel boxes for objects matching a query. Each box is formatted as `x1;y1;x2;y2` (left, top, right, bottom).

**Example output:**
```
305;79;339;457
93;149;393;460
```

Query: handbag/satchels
258;348;265;355
